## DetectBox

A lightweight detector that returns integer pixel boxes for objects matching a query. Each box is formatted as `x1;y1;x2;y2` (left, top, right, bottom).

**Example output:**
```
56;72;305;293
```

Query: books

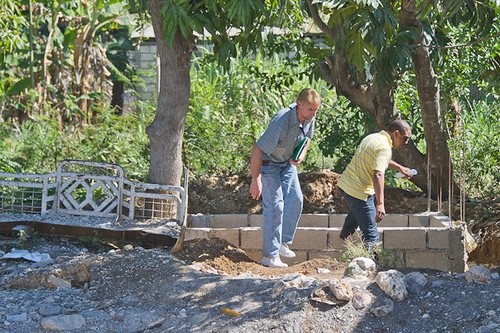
292;137;308;161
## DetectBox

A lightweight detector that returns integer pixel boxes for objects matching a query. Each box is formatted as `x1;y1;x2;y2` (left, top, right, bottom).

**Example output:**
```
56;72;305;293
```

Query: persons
337;119;413;265
249;87;321;268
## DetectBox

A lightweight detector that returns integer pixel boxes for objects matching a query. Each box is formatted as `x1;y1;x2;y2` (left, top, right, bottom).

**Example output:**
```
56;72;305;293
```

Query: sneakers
261;255;288;268
279;243;296;258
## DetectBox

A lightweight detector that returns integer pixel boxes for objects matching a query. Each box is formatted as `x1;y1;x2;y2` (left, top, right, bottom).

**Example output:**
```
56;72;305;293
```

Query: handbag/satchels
292;137;310;162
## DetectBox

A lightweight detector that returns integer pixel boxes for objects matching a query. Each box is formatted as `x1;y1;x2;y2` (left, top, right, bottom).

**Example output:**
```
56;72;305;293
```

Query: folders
292;136;308;161
